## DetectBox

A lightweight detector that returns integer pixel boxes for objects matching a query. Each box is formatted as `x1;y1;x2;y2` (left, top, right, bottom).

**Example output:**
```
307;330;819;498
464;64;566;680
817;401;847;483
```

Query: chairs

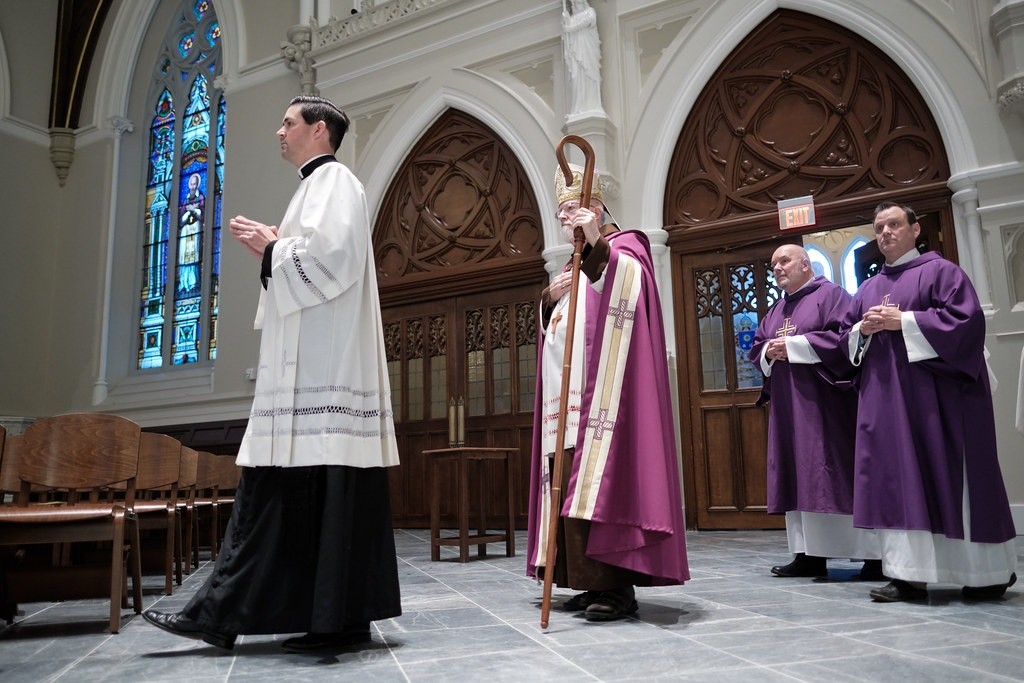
0;412;244;636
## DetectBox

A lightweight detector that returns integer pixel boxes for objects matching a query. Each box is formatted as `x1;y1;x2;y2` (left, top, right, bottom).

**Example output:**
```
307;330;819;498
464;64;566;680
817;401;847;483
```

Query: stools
422;446;522;563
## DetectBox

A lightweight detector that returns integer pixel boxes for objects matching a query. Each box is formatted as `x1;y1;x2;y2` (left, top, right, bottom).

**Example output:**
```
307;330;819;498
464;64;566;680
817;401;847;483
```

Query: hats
555;162;605;205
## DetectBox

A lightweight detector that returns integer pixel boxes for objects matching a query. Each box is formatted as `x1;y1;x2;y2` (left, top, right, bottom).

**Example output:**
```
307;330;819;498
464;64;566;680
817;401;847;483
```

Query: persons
748;242;883;579
839;200;1018;603
528;162;689;621
144;98;402;650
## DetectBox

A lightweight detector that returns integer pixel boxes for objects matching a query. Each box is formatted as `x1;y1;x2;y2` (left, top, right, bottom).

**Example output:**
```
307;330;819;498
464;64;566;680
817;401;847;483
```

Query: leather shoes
870;579;929;602
772;555;828;577
144;610;237;650
282;633;369;652
587;593;641;618
860;564;883;581
563;591;593;609
960;572;1017;600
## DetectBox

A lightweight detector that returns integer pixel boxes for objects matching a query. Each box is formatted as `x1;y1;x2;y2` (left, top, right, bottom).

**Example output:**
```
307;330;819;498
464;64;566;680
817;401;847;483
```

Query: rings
561;281;564;287
